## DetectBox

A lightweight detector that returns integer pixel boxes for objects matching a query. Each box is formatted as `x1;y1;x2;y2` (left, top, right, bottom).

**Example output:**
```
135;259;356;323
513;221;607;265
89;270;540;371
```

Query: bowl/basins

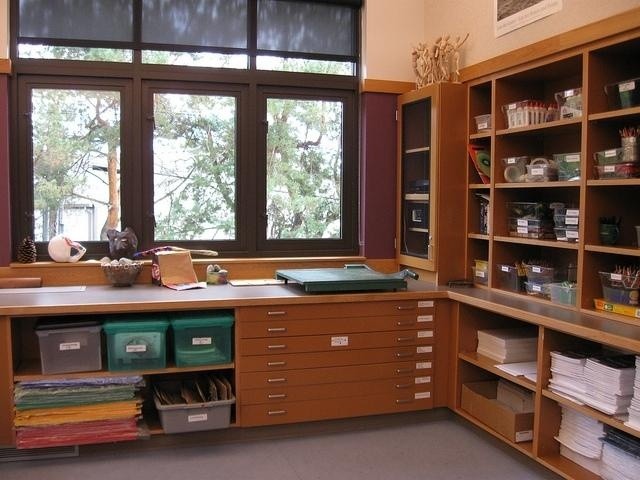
101;265;142;286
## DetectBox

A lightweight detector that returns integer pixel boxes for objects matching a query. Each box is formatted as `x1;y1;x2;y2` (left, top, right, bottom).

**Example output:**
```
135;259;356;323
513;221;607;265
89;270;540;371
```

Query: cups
601;223;621;245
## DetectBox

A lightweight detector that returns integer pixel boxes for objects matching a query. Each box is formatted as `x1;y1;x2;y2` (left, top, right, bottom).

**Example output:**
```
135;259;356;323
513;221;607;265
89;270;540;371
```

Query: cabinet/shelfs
462;30;640;329
391;82;468;270
238;301;436;429
4;308;238;452
448;301;640;480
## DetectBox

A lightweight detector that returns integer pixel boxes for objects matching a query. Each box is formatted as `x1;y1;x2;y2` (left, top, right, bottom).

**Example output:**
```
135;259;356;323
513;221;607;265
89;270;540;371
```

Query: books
467;326;640;480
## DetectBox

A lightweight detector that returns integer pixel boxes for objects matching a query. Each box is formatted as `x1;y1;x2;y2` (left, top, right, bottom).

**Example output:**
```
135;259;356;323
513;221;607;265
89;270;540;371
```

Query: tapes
504;166;521;182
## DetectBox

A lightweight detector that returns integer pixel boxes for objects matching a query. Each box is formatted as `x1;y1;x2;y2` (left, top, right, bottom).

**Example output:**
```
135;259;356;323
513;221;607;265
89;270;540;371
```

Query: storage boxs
152;382;237;436
460;380;534;444
35;310;238;375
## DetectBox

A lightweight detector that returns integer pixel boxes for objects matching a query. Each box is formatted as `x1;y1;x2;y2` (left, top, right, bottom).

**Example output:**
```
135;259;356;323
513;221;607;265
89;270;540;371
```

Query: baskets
501;93;564;129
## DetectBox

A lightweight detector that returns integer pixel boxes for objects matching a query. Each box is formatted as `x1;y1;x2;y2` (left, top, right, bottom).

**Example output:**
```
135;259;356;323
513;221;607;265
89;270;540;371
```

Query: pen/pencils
622;130;636;137
612;268;638;291
